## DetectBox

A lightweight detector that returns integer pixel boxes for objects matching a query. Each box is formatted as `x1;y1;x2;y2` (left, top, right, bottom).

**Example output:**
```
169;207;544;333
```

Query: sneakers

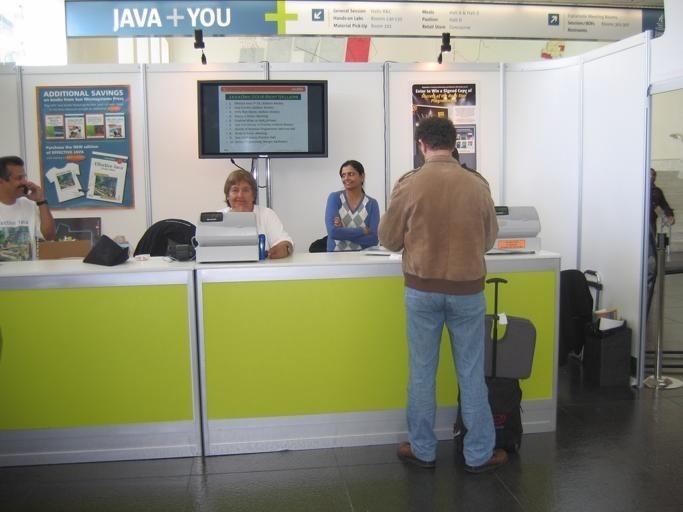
464;450;507;474
398;440;438;469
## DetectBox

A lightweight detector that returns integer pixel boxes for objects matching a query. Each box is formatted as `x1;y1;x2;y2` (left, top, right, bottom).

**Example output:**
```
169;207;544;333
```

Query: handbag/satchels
484;314;537;379
84;235;129;266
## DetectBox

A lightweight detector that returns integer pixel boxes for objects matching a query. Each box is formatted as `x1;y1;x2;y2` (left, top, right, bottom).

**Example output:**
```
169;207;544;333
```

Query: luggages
454;278;523;456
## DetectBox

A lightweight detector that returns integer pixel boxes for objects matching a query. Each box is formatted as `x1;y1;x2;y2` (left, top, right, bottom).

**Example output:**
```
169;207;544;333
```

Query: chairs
130;218;196;261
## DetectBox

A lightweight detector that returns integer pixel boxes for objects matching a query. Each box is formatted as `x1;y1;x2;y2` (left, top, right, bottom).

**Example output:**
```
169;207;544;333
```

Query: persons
216;170;294;260
0;156;57;262
646;168;676;320
378;115;509;473
452;147;489;184
324;160;380;253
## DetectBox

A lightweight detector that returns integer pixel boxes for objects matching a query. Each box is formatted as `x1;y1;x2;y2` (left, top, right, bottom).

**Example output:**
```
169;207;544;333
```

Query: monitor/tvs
196;80;328;159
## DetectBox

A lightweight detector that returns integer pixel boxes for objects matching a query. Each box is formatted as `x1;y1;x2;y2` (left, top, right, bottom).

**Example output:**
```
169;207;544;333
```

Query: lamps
192;29;205;60
437;35;453;66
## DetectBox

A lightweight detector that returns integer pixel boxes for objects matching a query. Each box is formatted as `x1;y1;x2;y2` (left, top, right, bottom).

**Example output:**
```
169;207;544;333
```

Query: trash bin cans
589;327;631;387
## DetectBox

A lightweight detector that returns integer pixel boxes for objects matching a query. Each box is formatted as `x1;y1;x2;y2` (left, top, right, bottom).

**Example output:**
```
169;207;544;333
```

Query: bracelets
286;246;291;256
36;199;49;206
